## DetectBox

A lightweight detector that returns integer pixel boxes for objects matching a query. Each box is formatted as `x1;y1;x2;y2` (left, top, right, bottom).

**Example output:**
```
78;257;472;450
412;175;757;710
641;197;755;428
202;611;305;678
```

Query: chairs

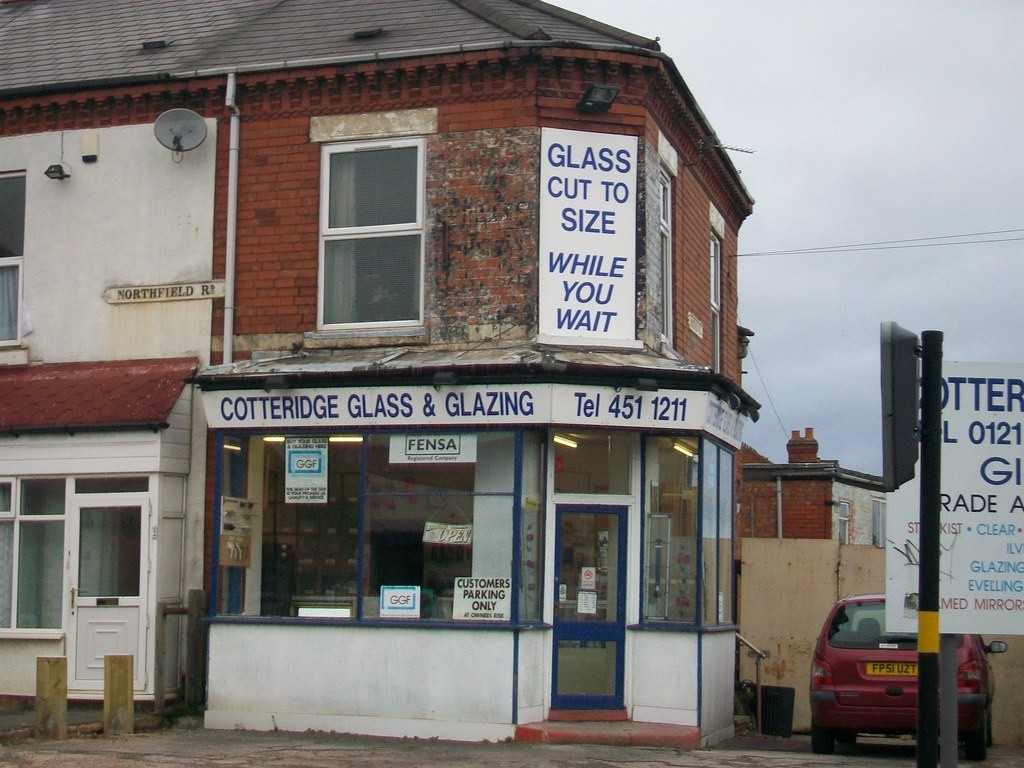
857;619;880;640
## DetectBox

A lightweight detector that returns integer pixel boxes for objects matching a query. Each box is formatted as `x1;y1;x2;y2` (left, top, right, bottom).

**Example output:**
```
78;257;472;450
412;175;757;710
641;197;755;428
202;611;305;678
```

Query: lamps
45;164;70;180
575;83;619;116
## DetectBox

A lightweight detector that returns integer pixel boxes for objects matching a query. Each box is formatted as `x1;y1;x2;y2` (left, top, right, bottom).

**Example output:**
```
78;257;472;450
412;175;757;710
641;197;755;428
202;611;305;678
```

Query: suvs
809;593;1008;761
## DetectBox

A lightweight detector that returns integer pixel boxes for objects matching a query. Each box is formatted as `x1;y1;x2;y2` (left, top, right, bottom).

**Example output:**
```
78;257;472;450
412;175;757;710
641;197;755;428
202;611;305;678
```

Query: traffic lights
880;321;919;492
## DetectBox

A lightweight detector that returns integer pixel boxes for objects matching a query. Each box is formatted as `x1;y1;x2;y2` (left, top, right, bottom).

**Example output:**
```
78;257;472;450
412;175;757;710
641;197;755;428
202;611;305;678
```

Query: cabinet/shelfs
267;468;359;594
